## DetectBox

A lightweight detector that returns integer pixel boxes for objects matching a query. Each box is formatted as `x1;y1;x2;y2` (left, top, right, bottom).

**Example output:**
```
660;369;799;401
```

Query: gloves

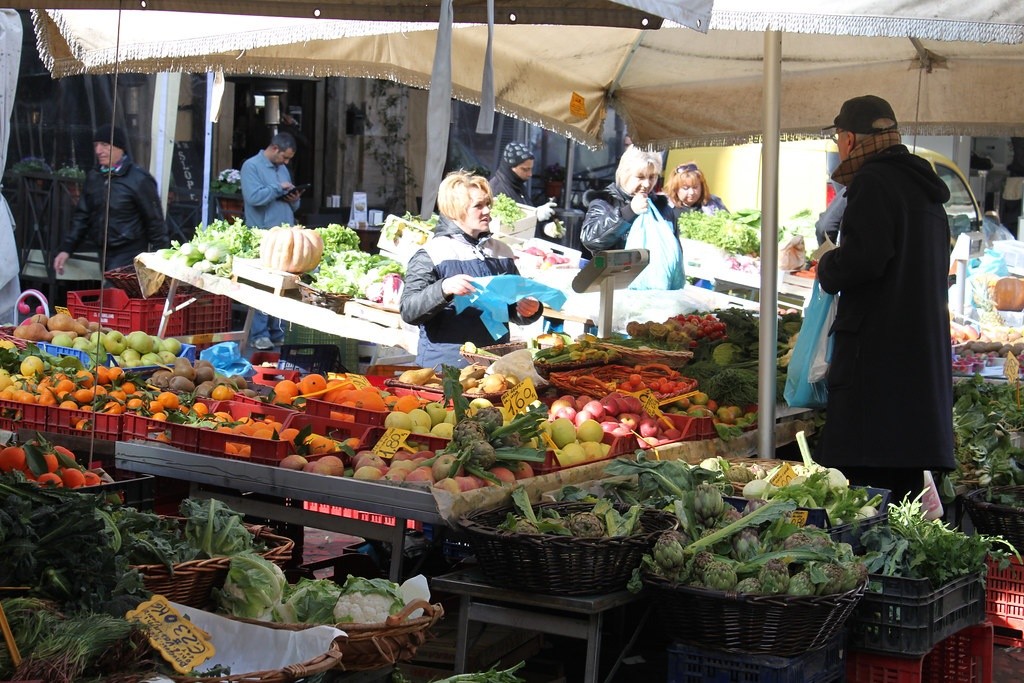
536;202;557;221
543;220;567;238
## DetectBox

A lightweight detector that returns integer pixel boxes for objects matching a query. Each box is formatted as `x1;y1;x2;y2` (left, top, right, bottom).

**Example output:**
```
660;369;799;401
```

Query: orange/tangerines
0;366;361;457
272;374;419;422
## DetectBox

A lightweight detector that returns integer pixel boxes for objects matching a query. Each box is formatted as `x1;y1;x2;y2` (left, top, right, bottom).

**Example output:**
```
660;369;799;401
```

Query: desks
429;565;646;683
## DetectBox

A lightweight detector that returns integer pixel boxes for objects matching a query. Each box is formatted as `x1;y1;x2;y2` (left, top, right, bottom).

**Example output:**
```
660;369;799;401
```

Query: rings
528;312;531;315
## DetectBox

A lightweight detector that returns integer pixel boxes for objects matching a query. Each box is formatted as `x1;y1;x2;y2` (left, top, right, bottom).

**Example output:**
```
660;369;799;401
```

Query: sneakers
273;337;284;347
250;337;276;349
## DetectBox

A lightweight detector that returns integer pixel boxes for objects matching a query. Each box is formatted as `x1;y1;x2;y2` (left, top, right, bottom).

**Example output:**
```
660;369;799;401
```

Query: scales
954;231;985;324
572;248;650;339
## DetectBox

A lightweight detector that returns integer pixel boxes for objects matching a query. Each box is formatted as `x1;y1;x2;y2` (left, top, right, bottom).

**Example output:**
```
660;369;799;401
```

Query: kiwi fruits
152;357;269;401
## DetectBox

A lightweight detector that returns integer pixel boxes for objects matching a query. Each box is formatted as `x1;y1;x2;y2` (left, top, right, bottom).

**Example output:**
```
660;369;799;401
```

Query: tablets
276;183;311;200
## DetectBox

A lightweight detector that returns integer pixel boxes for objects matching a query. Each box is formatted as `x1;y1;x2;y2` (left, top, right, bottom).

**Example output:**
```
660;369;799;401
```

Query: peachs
0;441;101;488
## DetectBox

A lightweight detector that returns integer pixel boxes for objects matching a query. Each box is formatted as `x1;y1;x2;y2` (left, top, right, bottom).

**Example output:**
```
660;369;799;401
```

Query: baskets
0;262;1024;683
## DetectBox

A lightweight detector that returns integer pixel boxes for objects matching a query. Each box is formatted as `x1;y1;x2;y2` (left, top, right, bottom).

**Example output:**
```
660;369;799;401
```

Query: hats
503;142;534;168
822;94;898;133
92;124;128;154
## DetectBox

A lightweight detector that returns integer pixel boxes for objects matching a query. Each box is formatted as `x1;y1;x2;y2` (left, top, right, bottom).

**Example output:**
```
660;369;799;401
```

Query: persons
241;131;305;349
818;95;957;501
399;168;543;373
657;163;729;217
54;123;170;289
580;144;677;256
489;141;567;242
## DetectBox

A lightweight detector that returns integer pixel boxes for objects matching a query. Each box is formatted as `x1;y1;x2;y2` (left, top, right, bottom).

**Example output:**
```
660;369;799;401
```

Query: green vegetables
686;307;800;408
939;372;1024;507
678;210;811;258
0;469;254;683
433;660;526;683
860;486;1023;591
389;192;526;245
152;216;404;294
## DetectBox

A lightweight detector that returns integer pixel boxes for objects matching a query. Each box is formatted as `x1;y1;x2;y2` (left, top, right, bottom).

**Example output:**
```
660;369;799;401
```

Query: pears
399;365;521;394
12;314;105;341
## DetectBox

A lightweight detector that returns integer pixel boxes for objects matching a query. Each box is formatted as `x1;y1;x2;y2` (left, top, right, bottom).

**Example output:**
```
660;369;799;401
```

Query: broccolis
220;561;395;625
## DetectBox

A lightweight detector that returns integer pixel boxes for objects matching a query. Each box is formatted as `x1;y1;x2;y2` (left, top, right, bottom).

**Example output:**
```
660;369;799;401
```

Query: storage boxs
986;555;1024;647
666;632;846;683
844;621;994;683
0;290;758;532
722;486;892;557
844;572;986;657
218;198;244;226
376;200;582;278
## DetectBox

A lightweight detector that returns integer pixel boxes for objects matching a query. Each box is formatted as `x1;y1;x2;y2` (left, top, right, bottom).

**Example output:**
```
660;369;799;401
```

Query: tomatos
668;313;727;346
665;393;755;426
616;374;688;399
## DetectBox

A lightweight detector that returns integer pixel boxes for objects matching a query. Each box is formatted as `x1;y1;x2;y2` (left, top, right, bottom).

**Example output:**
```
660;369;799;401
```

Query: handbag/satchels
782;275;841;411
623;199;686;289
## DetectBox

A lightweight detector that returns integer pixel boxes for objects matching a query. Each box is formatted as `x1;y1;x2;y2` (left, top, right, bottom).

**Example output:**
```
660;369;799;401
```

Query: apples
547;393;681;447
279;451;533;491
385;397;611;466
51;329;182;367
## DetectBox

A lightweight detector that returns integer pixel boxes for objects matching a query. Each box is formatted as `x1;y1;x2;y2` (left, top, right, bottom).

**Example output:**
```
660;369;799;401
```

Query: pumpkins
260;224;324;273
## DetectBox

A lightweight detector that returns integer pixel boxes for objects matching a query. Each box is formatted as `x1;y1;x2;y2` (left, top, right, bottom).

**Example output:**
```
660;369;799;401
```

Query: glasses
830;129;846;145
675;164;699;175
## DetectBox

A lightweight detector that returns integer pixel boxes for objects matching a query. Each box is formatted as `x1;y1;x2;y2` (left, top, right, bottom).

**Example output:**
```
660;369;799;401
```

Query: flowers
9;155;87;179
210;169;243;195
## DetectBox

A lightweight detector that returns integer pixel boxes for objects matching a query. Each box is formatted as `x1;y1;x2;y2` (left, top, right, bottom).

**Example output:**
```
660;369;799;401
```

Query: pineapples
971;277;1004;327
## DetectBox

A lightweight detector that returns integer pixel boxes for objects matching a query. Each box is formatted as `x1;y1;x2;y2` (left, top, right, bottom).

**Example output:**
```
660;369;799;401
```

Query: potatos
961;331;1024;358
